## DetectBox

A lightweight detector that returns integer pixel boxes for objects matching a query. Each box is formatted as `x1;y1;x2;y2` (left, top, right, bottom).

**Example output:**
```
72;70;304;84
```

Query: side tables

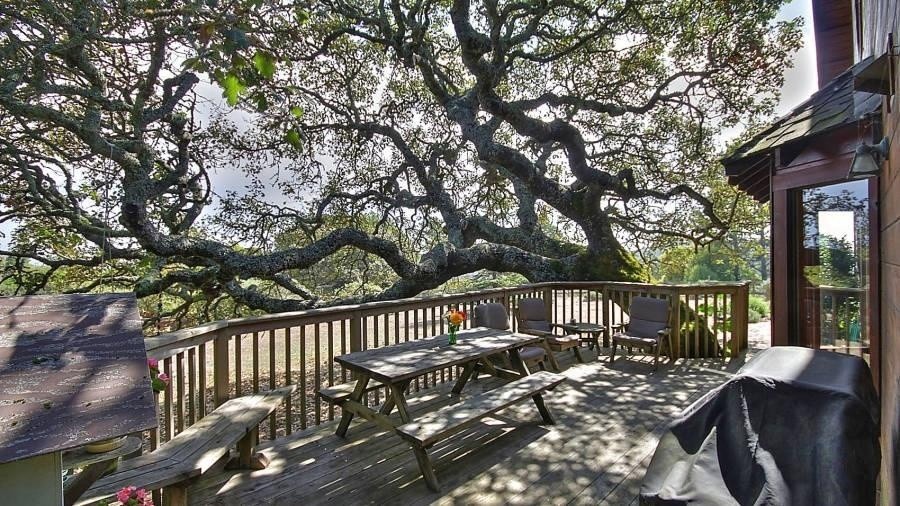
565;323;605;355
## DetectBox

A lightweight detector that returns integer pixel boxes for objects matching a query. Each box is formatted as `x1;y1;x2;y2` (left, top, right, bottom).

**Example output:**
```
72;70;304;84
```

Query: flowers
441;308;469;342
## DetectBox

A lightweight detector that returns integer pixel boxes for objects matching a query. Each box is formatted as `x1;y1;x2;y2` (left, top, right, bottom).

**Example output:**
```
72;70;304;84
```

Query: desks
334;326;557;491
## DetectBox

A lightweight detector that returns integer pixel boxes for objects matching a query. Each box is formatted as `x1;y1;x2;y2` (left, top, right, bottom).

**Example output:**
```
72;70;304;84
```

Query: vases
448;324;457;345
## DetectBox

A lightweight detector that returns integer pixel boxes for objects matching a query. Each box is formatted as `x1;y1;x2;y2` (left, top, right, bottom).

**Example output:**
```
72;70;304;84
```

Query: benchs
65;385;297;506
318;369;568;491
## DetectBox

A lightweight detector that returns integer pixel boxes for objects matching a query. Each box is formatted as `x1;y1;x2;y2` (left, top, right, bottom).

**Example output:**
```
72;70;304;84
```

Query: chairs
475;298;584;372
610;296;674;373
